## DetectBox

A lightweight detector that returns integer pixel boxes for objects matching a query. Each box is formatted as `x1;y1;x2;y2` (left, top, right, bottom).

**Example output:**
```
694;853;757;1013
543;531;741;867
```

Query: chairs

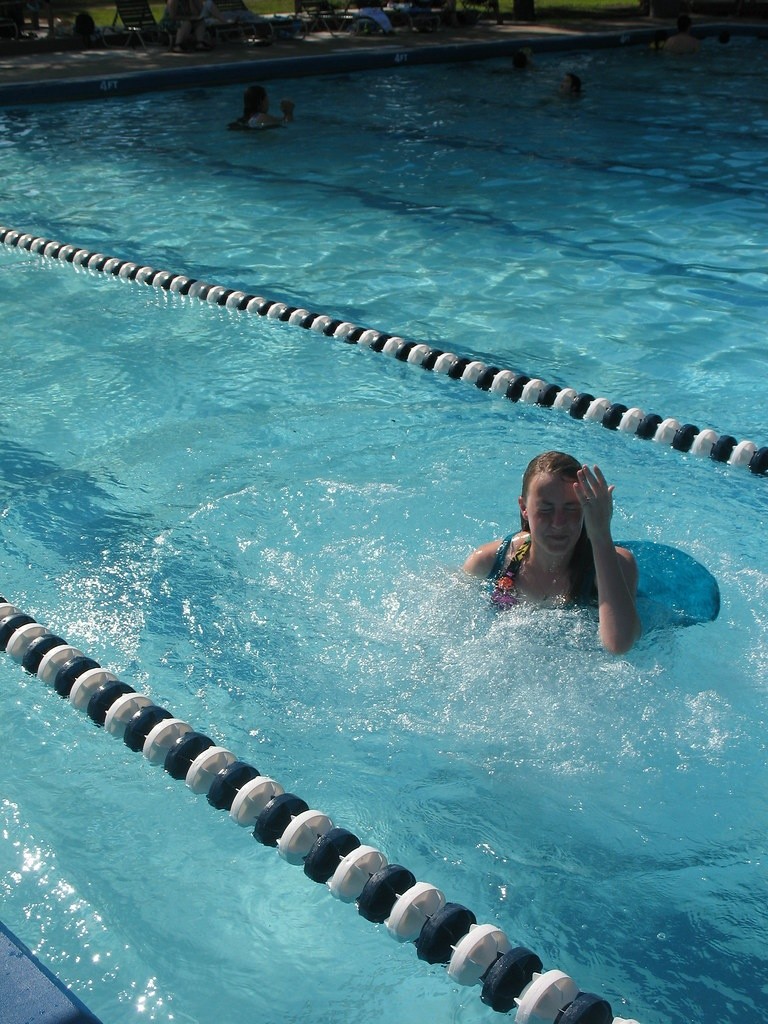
0;0;495;56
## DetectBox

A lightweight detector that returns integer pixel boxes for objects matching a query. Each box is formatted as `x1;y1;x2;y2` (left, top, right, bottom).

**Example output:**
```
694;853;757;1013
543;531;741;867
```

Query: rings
582;502;588;506
586;496;595;500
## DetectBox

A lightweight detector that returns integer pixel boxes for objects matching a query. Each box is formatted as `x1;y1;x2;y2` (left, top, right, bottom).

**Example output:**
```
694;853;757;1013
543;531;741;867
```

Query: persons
463;451;641;653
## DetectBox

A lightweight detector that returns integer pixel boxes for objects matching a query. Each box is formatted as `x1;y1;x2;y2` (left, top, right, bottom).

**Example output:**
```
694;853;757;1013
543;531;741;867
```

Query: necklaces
529;565;556;599
